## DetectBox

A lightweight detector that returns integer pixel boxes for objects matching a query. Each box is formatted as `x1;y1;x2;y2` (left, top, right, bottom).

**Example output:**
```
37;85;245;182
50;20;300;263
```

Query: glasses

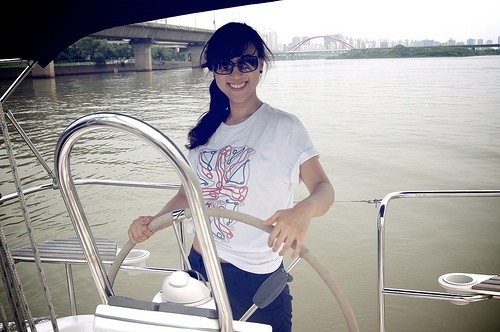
212;55;261;75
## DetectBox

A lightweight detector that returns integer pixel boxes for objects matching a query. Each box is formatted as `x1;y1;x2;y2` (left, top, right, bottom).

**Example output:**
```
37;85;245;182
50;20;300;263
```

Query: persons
127;21;335;330
